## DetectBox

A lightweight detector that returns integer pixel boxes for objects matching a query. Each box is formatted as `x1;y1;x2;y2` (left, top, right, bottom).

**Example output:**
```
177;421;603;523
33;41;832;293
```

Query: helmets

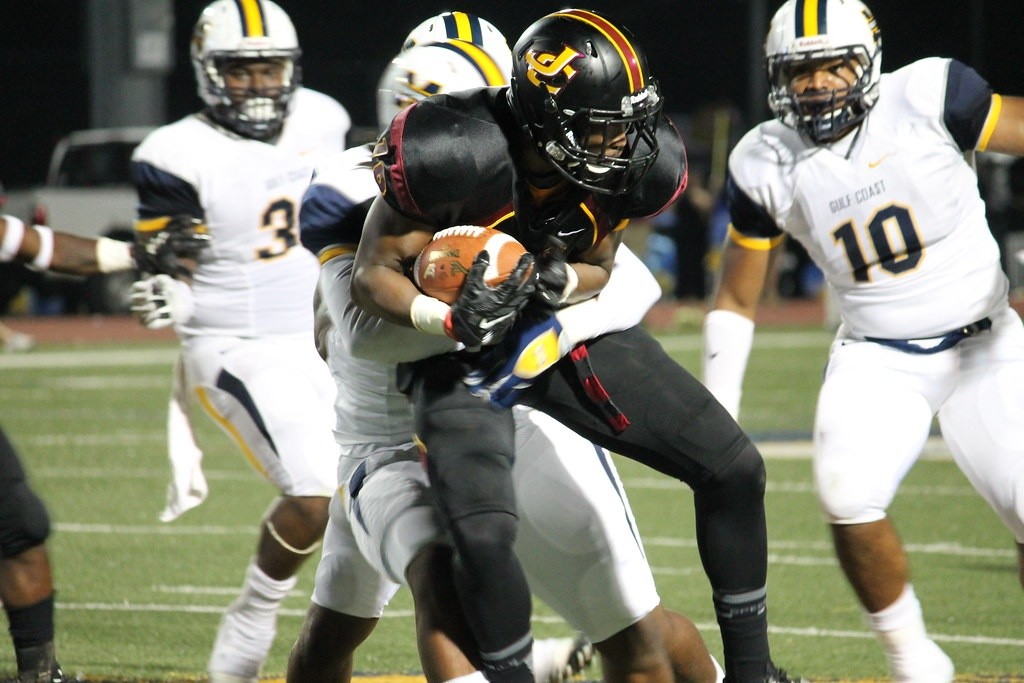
394;10;512;87
766;0;883;145
511;7;661;194
185;1;302;144
374;48;482;130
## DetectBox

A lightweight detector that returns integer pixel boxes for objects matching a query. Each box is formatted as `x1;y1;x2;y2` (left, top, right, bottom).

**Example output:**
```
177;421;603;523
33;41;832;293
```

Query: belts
863;314;991;355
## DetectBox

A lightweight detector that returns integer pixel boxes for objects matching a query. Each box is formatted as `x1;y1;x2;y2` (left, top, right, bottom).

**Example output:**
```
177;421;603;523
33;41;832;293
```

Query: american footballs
405;225;528;306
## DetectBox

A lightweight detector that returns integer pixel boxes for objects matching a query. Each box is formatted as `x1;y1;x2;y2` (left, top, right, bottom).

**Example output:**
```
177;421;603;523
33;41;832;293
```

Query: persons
699;0;1024;683
126;0;352;683
0;214;213;683
286;9;809;683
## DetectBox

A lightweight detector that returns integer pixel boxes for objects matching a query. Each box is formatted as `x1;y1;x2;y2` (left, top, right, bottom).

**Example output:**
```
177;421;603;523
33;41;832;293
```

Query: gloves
408;249;540;353
128;213;213;276
527;232;571;311
119;274;202;337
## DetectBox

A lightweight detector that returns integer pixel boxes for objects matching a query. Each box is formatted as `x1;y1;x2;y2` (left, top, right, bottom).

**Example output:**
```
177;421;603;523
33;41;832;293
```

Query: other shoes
209;597;272;683
516;628;600;683
896;645;953;683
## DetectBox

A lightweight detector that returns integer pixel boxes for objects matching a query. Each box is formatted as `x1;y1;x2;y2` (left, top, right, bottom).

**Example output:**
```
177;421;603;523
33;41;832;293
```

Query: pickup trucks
0;125;157;312
